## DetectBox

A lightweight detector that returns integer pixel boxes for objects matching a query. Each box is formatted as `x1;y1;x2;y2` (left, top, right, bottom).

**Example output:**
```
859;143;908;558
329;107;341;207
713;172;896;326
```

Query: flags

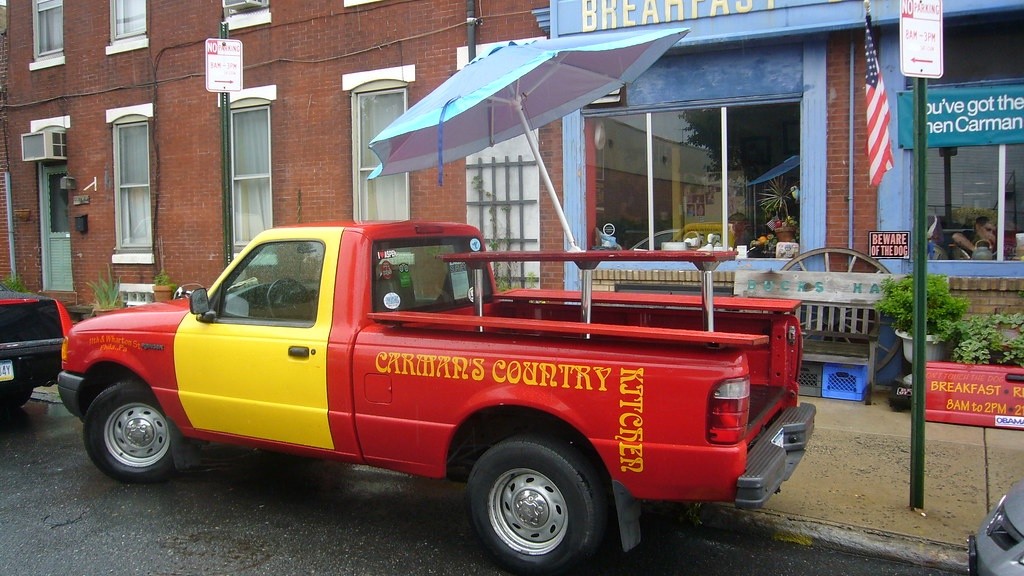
865;14;894;187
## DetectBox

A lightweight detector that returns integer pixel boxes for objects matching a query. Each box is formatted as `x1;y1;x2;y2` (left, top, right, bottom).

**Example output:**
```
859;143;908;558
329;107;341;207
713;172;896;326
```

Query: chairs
378;270;416;309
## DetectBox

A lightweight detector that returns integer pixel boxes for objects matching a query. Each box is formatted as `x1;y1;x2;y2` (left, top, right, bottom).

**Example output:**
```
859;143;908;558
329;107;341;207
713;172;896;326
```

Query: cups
1016;233;1024;261
737;245;747;258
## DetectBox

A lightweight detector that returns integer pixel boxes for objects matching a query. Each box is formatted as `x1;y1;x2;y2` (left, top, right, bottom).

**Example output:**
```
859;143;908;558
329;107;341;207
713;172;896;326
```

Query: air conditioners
20;129;68;162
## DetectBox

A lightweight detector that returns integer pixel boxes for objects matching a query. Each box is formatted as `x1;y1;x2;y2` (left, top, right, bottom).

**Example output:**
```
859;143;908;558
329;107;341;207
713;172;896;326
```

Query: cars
966;480;1024;576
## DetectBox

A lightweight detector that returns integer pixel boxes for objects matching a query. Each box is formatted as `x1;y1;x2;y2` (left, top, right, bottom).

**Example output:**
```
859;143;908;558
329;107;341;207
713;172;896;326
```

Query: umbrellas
747;155;800;187
366;27;691;245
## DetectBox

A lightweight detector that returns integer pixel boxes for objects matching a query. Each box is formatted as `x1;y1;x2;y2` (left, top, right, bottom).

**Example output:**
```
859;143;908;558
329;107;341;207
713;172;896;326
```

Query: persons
927;215;949;259
952;216;996;259
728;213;754;258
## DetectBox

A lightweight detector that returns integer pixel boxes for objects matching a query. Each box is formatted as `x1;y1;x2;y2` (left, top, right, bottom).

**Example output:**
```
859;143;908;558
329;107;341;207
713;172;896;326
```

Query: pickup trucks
56;217;818;575
0;282;74;419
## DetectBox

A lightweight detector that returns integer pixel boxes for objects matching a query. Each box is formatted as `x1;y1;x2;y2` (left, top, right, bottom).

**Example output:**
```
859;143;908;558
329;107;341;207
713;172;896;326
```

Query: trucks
630;222;750;257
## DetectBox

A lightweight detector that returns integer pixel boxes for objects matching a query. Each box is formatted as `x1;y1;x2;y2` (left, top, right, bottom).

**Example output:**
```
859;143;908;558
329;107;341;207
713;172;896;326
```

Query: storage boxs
794;361;870;400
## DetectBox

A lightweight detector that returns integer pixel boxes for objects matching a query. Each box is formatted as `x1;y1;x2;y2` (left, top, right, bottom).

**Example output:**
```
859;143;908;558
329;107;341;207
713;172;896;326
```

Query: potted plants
755;180;795;242
88;263;126;317
878;274;971;365
153;267;177;303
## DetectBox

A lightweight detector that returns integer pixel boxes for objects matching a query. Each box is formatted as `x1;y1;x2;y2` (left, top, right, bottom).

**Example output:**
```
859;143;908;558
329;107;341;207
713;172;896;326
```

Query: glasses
982;226;995;235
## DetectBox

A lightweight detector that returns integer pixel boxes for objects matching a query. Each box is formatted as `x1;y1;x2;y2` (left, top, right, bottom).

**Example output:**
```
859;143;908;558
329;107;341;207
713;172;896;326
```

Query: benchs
733;272;903;403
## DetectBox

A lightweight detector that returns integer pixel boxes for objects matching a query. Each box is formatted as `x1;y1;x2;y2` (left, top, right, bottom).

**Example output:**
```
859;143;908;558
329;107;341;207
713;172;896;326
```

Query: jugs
973;239;997;259
